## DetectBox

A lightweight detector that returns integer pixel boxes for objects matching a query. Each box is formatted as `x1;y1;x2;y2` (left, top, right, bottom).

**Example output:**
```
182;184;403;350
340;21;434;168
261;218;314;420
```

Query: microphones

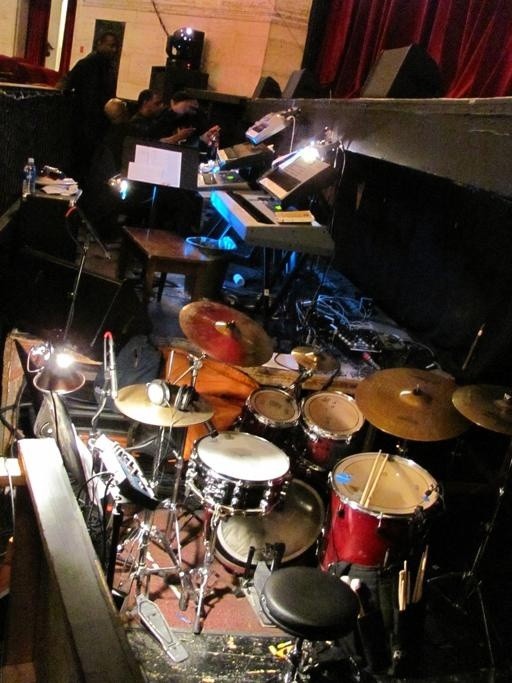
104;331;119;399
75;207;112;260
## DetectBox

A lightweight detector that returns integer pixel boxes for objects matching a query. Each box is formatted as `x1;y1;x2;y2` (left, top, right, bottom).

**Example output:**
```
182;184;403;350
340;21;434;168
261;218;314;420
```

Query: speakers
281;68;319;99
252;76;282;98
360;44;441;98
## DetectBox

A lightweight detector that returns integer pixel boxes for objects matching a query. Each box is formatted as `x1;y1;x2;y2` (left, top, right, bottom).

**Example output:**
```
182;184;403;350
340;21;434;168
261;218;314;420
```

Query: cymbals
180;301;275;365
453;383;511;434
115;382;215;426
292;346;340;371
355;367;474;441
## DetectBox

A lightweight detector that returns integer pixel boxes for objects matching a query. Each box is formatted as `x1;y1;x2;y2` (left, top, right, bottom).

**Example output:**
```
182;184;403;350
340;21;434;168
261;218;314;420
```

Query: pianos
211;189;334;257
216;143;275;170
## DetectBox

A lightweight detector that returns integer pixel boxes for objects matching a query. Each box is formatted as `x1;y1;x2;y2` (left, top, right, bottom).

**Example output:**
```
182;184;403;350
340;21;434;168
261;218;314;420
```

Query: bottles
41;165;65;181
21;157;38;196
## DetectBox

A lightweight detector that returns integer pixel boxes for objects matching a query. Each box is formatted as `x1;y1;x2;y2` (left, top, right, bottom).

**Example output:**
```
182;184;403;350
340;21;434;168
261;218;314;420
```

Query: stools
120;226;212;323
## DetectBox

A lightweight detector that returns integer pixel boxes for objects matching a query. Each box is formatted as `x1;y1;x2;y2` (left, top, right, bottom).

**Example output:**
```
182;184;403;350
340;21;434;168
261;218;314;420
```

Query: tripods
125;185;180;288
425;460;512;667
117;427;231;636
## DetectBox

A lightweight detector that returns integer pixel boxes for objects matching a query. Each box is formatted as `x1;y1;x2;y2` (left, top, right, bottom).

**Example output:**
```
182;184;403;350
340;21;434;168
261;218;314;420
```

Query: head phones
146;379;201;413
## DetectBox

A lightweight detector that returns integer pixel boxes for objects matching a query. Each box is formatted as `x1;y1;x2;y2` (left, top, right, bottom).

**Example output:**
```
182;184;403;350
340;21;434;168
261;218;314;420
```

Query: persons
130;89;165;134
65;31;125;164
158;87;197;150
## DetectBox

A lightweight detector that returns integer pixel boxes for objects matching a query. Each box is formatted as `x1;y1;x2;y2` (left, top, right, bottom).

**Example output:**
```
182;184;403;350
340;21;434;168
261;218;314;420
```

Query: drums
315;452;442;575
300;391;364;472
212;478;327;575
186;431;292;515
233;385;301;452
